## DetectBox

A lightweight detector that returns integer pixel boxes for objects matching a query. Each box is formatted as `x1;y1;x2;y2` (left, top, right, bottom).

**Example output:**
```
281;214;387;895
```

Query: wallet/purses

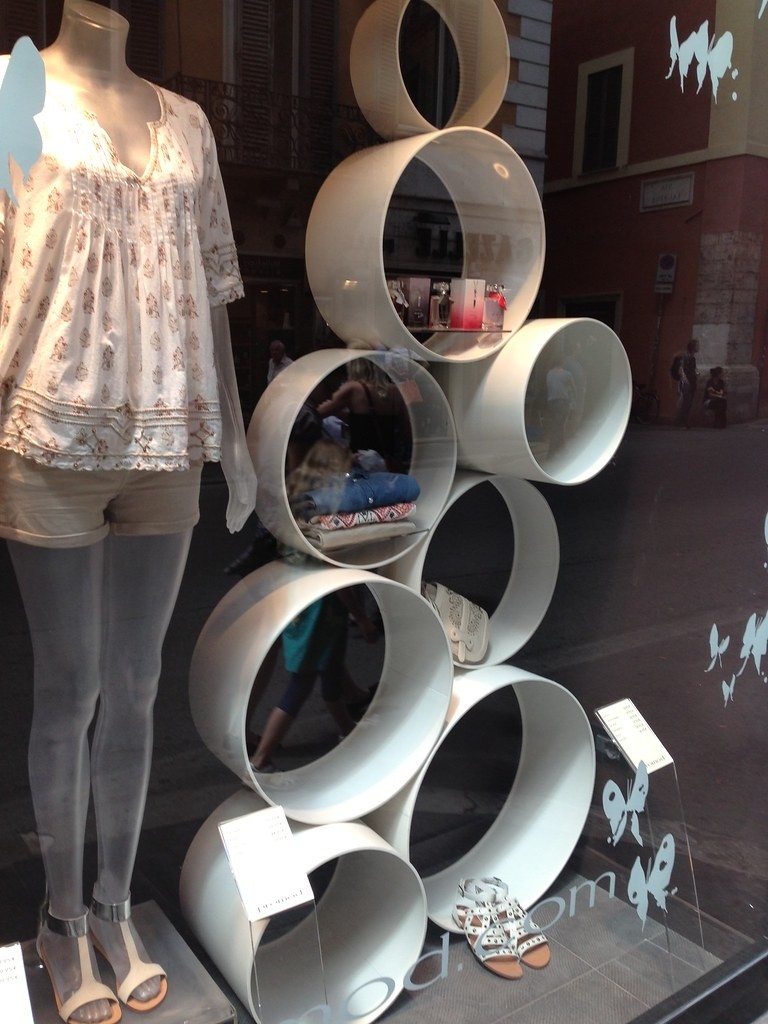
293;470;421;548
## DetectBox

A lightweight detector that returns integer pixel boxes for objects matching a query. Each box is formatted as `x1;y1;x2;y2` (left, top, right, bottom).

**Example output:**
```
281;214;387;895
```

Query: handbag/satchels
422;580;490;663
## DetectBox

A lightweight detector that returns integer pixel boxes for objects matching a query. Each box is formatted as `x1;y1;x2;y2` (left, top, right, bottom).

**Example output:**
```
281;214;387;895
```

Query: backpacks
671;355;683;381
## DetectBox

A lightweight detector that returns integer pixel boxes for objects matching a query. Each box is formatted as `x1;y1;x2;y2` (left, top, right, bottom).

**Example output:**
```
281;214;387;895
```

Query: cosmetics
429;282;454;329
387;280;406;324
482;284;505;329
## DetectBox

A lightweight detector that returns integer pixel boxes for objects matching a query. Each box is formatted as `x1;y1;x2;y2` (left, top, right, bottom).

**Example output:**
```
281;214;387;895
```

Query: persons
678;339;700;430
545;355;577;461
225;329;426;771
0;0;261;1024
703;365;728;429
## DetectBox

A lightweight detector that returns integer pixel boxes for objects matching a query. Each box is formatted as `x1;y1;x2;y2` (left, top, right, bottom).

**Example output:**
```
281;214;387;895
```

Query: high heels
346;690;374;721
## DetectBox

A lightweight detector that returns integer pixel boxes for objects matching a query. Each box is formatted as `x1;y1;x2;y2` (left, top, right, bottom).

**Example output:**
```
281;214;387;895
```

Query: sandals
87;881;168;1012
451;875;551;980
36;883;122;1024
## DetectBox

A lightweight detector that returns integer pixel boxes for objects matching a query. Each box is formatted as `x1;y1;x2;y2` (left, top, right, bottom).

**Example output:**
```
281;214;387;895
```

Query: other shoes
241;761;287;789
337;720;358;743
